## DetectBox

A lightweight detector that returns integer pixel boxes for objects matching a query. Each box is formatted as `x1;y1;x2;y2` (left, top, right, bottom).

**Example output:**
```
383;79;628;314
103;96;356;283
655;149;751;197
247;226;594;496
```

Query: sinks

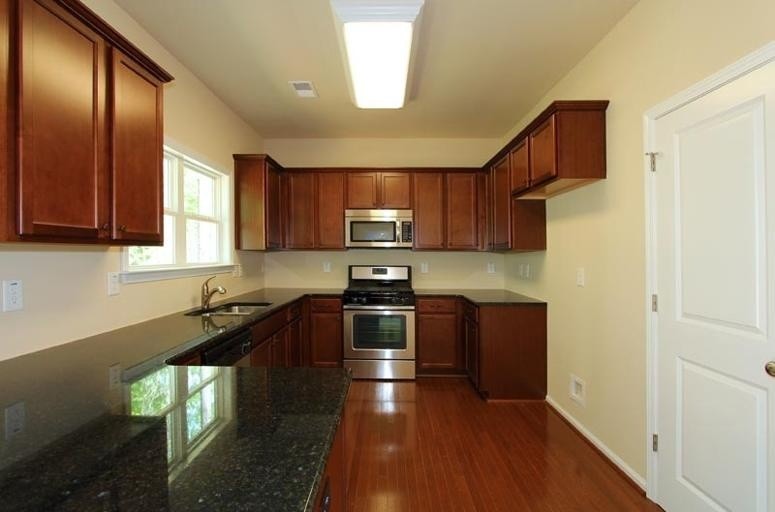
184;300;274;317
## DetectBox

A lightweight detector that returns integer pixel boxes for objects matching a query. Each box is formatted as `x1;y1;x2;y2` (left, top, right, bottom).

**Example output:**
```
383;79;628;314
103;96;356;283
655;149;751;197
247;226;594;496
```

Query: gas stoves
343;284;415;305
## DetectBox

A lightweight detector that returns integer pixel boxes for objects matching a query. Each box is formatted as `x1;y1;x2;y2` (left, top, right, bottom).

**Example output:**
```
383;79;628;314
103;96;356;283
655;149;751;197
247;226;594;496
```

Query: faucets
199;312;227;335
198;275;226;309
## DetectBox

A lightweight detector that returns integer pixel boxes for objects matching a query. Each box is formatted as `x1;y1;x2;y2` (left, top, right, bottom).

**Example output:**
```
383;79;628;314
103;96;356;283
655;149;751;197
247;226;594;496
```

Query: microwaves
344;208;413;249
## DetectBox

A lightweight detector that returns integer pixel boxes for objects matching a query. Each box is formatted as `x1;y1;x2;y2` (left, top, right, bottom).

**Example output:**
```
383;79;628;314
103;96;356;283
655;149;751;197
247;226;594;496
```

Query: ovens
342;306;416;381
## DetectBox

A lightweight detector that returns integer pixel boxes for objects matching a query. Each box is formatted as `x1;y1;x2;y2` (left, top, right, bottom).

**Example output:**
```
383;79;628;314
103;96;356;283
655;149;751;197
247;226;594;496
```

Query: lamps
328;0;428;110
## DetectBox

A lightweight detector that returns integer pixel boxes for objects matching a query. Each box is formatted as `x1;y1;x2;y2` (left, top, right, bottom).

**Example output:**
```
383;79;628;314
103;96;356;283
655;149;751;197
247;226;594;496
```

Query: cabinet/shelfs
463;288;548;404
297;401;353;510
414;295;466;378
305;289;345;369
345;167;412;210
232;155;284;252
477;148;546;253
284;167;347;251
0;0;178;248
171;350;200;366
510;100;609;200
410;167;479;252
249;295;305;369
